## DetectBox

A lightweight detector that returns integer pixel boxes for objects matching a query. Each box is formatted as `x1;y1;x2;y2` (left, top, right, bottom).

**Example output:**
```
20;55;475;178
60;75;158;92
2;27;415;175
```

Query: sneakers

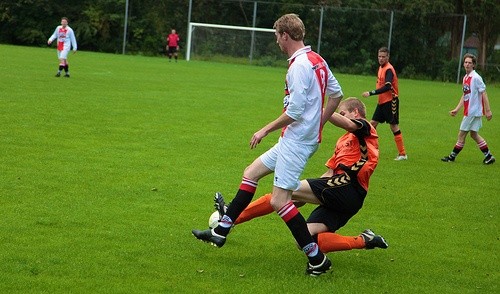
305;255;332;278
191;229;226;248
394;155;407;161
483;154;495;165
213;192;227;222
359;229;388;250
441;155;455;162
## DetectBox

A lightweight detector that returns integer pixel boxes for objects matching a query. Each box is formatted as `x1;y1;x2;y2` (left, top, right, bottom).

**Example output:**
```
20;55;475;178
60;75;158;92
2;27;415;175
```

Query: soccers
209;210;234;235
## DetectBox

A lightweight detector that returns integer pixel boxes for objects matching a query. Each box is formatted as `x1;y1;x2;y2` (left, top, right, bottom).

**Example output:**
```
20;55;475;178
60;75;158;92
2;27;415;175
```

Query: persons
47;17;77;78
191;13;333;279
361;47;407;161
214;96;389;253
166;29;180;63
441;53;496;165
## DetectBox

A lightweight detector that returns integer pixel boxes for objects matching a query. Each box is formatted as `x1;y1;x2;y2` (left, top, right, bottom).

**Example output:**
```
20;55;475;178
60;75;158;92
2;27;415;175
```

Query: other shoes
55;73;60;77
64;73;70;77
